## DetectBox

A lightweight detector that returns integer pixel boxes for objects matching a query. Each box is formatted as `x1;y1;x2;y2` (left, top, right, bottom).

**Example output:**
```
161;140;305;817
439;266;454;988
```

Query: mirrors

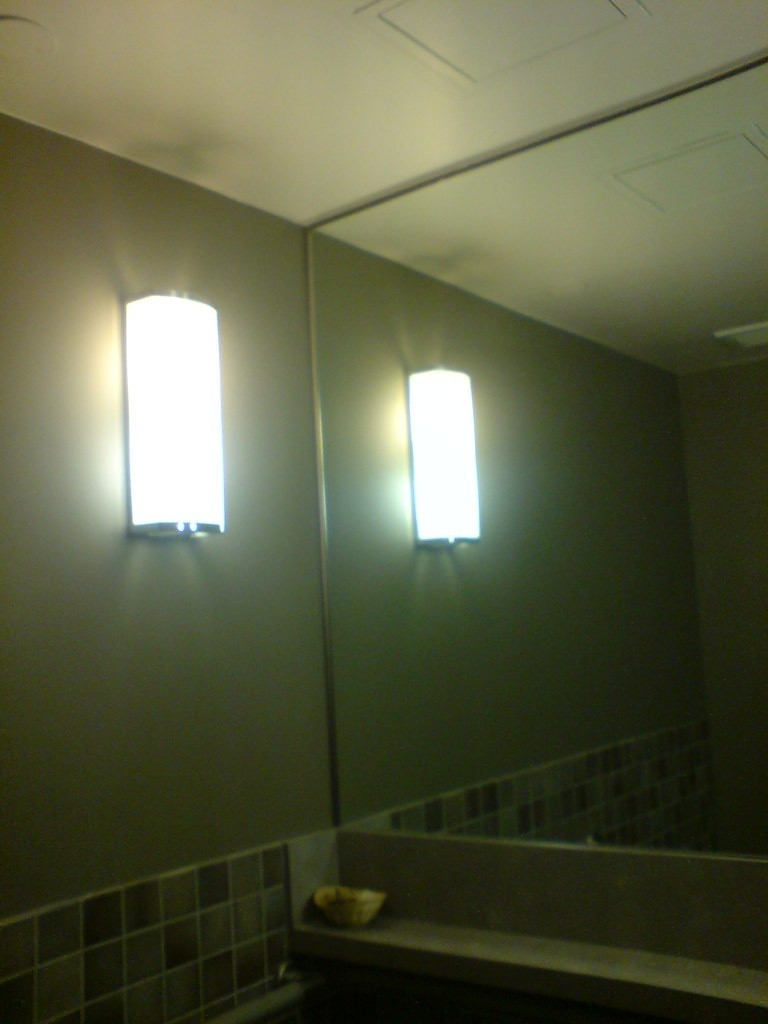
301;54;768;862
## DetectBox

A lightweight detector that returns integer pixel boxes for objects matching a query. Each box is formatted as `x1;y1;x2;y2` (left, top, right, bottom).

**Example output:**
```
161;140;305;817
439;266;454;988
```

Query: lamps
406;366;483;549
118;288;228;542
